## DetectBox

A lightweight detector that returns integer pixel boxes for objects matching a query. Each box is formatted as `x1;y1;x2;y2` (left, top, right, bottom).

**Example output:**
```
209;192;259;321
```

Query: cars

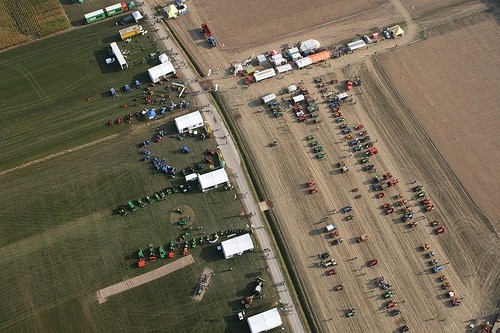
86;17;466;333
